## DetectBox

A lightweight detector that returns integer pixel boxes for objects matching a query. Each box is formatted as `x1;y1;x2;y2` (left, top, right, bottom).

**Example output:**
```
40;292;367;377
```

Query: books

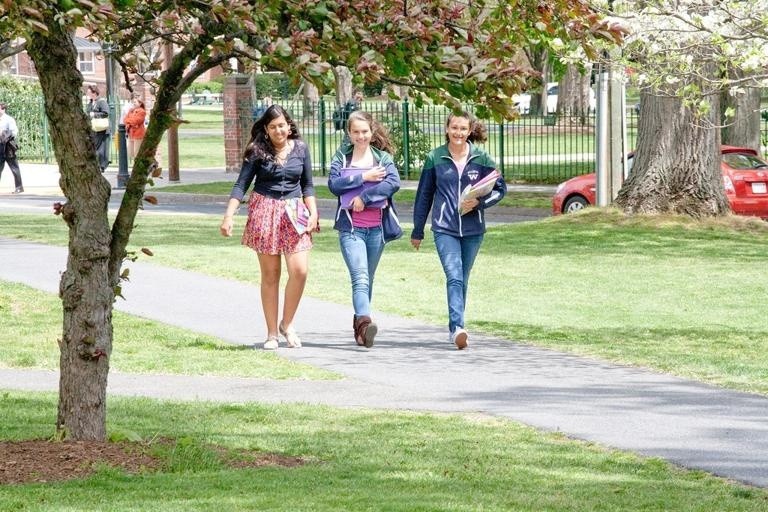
457;168;501;217
339;164;388;209
284;199;320;236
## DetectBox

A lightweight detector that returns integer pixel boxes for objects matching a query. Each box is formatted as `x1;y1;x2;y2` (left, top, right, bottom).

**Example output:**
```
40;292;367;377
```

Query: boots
352;314;378;348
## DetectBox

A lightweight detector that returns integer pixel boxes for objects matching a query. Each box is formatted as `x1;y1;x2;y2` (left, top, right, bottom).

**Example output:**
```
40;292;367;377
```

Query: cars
512;82;595;115
552;145;767;219
634;101;640;113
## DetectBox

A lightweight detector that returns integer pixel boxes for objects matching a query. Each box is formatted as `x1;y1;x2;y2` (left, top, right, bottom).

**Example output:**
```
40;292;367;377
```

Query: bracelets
221;215;235;220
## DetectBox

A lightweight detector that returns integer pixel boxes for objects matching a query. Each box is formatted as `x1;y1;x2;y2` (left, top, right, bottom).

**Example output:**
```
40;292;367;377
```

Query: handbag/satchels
90;117;109;132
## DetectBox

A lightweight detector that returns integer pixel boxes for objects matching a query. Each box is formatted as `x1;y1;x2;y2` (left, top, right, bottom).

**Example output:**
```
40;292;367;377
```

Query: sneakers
448;327;469;350
278;319;302;348
263;336;279;350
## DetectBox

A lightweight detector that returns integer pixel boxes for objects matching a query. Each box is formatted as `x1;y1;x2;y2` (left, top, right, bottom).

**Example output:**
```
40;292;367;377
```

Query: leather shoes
12;187;24;194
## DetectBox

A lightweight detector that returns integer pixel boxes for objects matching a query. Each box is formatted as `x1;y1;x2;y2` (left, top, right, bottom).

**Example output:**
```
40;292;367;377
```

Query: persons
409;107;508;353
85;85;109;174
342;91;363;135
0;103;25;194
122;98;146;158
253;96;272;122
120;92;141;124
327;109;405;348
220;104;318;352
